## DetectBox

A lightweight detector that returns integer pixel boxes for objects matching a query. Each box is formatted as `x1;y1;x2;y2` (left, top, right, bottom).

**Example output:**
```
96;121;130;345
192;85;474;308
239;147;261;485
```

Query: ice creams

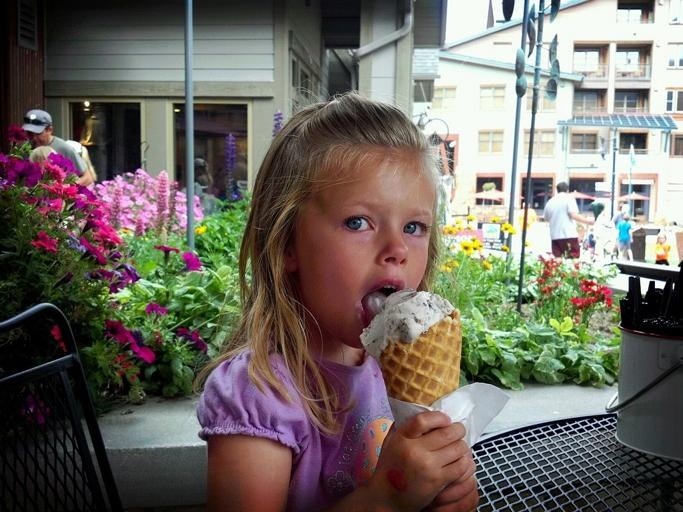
359;286;463;409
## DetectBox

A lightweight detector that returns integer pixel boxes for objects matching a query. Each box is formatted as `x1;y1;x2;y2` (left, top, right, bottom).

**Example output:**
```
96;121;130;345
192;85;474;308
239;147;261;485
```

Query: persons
192;87;480;512
17;107;98;189
542;181;672;266
65;140;97;182
29;145;57;166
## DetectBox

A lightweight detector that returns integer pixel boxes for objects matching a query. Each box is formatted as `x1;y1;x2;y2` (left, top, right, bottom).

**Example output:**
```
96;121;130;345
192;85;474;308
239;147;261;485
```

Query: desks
469;412;683;512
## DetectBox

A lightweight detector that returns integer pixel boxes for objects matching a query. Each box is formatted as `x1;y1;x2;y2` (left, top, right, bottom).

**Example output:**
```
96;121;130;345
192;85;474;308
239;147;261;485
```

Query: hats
194;158;205;167
20;110;54;135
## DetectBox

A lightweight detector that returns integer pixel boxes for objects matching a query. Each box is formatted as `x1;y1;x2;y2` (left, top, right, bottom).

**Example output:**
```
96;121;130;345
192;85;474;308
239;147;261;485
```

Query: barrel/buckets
604;319;683;462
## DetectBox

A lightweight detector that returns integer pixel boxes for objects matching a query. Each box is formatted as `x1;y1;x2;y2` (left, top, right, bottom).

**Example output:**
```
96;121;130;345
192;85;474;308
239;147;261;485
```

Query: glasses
23;116;48;125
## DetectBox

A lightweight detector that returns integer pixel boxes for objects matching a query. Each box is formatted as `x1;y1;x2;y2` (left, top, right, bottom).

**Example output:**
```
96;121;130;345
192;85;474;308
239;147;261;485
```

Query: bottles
618;274;681;335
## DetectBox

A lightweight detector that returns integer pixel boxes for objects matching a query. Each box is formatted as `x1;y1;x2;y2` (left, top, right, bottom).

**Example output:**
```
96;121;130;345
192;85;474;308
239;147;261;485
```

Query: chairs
0;302;124;512
